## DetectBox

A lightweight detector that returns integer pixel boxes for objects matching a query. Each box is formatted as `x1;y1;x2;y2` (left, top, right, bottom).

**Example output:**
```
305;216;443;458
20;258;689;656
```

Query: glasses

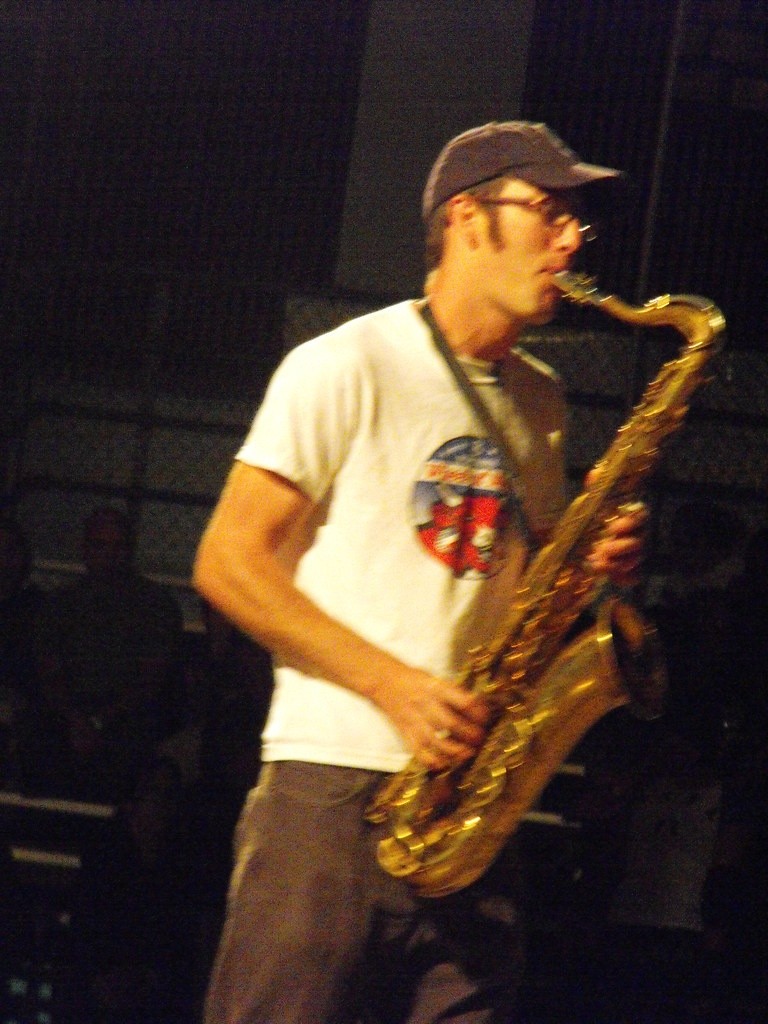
476;194;598;242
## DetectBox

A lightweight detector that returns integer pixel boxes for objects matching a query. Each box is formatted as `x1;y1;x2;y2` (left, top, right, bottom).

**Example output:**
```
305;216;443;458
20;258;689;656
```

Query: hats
421;121;621;218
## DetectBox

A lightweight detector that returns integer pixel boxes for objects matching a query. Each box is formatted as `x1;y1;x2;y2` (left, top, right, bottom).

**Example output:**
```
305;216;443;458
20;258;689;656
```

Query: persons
191;119;648;1024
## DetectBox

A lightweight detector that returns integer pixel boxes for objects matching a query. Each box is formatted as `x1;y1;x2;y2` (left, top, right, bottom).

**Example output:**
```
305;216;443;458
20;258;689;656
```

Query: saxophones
363;270;724;898
